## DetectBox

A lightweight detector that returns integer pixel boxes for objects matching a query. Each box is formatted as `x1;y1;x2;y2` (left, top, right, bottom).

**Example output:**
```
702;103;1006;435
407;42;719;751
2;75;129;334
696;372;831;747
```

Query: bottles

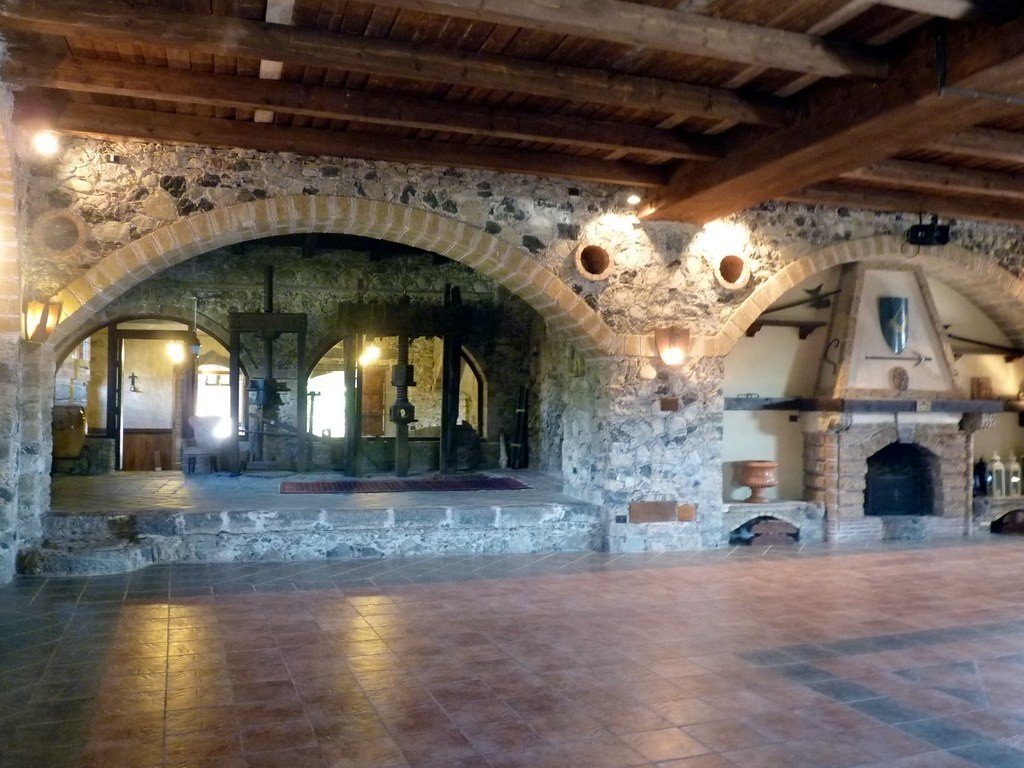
1005;448;1023;498
990;451;1005;497
973;456;992;497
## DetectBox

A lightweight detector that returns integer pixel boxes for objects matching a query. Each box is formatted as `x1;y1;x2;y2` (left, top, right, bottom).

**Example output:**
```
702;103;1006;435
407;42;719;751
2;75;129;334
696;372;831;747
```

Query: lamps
187;331;202;360
19;294;64;345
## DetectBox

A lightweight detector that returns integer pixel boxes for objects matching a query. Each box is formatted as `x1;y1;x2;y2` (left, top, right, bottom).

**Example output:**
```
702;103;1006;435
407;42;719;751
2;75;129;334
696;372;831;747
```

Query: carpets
281;477;533;495
